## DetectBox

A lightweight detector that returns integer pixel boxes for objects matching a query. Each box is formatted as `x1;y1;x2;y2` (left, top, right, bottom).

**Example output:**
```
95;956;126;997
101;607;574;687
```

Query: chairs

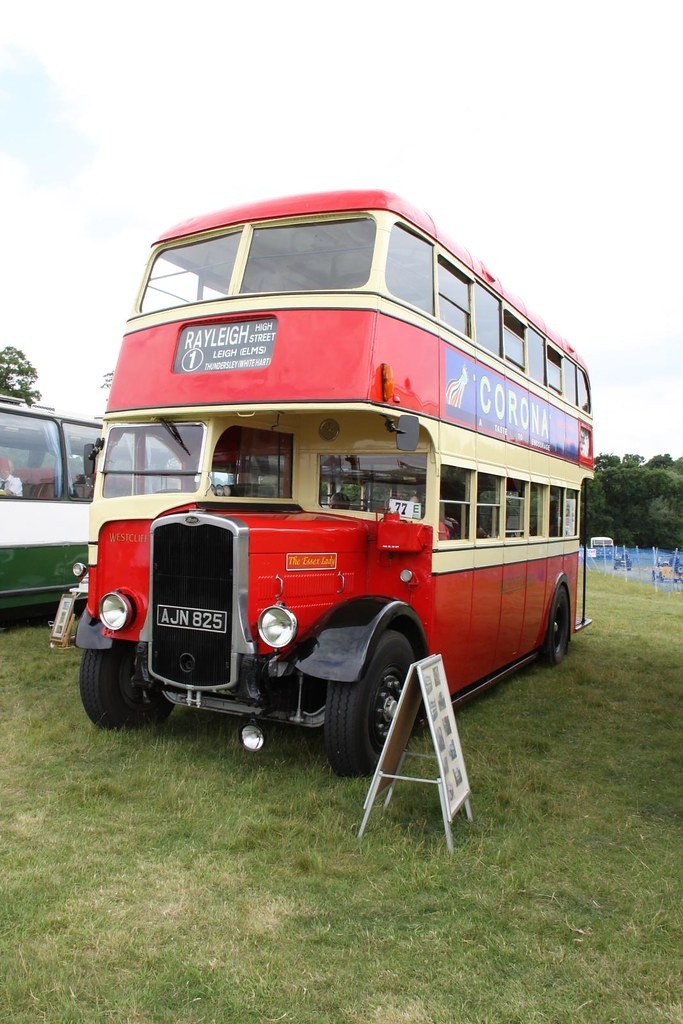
31;467;54;498
13;469;33;497
111;476;130;497
0;454;13;481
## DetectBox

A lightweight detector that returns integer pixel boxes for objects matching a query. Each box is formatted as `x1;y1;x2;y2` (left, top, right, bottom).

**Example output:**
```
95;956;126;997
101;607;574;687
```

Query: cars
580;547;596;558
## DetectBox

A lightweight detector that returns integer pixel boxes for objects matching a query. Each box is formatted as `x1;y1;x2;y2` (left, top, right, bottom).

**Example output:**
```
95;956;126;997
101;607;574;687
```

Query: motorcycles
651;555;683;584
613;554;632;571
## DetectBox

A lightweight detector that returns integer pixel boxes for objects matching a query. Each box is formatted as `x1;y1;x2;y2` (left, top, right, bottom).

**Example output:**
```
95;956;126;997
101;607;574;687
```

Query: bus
78;191;597;778
0;392;277;622
590;536;614;562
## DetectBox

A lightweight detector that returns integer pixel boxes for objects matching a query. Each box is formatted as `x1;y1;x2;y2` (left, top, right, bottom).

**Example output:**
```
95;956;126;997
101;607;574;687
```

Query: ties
0;481;6;490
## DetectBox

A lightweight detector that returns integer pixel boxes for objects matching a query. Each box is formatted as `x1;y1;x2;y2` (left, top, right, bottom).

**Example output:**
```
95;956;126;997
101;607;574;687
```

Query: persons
328;492;351;510
0;456;23;496
